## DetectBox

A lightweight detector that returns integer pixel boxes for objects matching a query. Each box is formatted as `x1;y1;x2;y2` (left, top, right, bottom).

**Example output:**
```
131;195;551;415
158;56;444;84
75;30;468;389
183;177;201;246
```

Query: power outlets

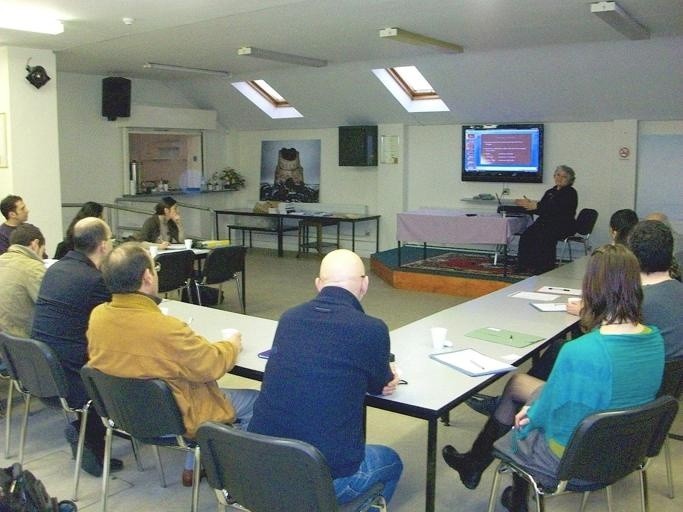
501;188;509;195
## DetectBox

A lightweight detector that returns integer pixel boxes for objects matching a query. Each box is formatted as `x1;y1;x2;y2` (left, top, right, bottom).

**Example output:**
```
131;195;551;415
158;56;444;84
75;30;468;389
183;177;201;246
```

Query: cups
431;326;448;350
568;297;582;306
221;328;237;340
184;238;193;249
163;183;169;191
149;245;157;256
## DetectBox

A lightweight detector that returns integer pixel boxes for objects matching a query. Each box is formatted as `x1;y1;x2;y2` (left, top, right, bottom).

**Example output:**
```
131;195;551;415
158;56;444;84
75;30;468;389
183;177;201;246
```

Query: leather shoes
464;393;498;416
101;458;123;470
182;468;205;487
63;421;102;477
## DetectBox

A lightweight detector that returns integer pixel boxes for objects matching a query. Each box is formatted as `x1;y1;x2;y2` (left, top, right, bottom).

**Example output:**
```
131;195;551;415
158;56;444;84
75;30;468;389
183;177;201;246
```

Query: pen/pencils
548;288;570;291
470;360;485;369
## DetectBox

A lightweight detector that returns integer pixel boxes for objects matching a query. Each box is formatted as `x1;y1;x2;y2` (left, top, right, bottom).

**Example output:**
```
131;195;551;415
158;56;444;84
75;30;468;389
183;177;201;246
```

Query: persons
642;212;683;282
1;194;185;478
464;219;683;417
247;249;403;511
515;165;578;275
610;209;681;281
442;244;665;511
86;246;260;487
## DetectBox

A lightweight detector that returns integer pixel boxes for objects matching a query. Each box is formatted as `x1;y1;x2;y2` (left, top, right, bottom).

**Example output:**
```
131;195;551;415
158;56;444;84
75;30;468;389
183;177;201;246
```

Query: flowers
220;164;244;190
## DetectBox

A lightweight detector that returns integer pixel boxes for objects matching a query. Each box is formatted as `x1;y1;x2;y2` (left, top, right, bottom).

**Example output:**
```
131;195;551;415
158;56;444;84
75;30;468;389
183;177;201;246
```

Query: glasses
104;234;115;243
152;261;161;273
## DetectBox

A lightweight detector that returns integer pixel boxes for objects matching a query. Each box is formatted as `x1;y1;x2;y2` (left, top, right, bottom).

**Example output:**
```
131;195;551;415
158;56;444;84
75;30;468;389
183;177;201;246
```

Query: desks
212;203;380;265
394;209;528;268
31;236;664;512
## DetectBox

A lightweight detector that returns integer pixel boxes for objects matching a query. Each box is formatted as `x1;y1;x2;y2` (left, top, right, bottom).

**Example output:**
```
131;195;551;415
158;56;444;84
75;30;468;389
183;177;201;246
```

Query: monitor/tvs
462;124;544;183
339;126;377;166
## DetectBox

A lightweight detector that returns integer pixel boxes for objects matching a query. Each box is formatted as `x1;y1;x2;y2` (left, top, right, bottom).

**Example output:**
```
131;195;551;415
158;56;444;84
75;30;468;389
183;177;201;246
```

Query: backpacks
0;462;77;512
181;285;224;306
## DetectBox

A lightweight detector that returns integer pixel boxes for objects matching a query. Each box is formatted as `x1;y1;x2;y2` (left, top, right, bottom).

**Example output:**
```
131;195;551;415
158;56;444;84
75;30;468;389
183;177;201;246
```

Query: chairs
5;330;171;492
0;333;67;460
188;421;389;512
77;364;207;512
151;245;195;302
485;394;678;512
604;347;682;512
199;244;247;316
554;208;598;269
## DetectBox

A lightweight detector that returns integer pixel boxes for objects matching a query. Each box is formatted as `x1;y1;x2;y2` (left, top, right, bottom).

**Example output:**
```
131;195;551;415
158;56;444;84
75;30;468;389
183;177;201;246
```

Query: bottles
200;175;207;191
130;180;136;195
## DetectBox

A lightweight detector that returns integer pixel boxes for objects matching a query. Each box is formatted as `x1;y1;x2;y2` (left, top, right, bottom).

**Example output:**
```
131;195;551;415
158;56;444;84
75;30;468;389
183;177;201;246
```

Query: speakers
102;77;131;117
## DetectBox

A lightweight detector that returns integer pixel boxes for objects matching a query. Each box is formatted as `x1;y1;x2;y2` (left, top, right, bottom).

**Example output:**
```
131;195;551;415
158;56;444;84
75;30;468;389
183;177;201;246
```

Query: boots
442;416;514;490
501;472;528;512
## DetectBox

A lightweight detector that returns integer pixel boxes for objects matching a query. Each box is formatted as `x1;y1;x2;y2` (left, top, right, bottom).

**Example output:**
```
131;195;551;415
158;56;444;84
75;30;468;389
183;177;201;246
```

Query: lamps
589;1;651;43
237;44;328;69
142;58;235;81
377;26;465;57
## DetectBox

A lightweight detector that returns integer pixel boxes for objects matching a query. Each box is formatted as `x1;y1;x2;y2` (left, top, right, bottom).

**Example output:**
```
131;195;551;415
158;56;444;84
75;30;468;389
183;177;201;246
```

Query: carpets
401;249;536;280
